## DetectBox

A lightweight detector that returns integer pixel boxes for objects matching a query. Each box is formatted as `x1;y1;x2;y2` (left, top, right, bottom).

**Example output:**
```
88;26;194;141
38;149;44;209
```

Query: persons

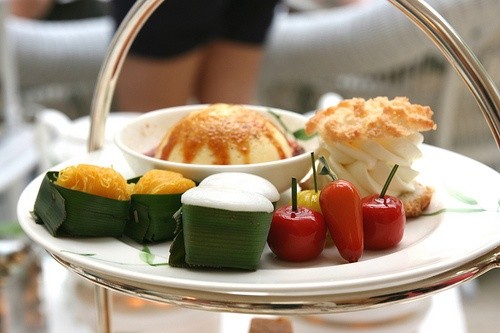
9;0;281;114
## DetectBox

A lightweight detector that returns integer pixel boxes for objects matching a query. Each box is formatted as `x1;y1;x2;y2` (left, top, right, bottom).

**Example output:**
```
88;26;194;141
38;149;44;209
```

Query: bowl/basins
113;104;317;192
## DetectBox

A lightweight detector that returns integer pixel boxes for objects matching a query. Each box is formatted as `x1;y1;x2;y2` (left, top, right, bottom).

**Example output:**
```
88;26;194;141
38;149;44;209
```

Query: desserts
30;95;438;271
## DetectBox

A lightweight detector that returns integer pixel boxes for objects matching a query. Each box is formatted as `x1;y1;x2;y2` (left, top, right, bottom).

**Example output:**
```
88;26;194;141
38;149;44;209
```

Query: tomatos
317;156;363;263
